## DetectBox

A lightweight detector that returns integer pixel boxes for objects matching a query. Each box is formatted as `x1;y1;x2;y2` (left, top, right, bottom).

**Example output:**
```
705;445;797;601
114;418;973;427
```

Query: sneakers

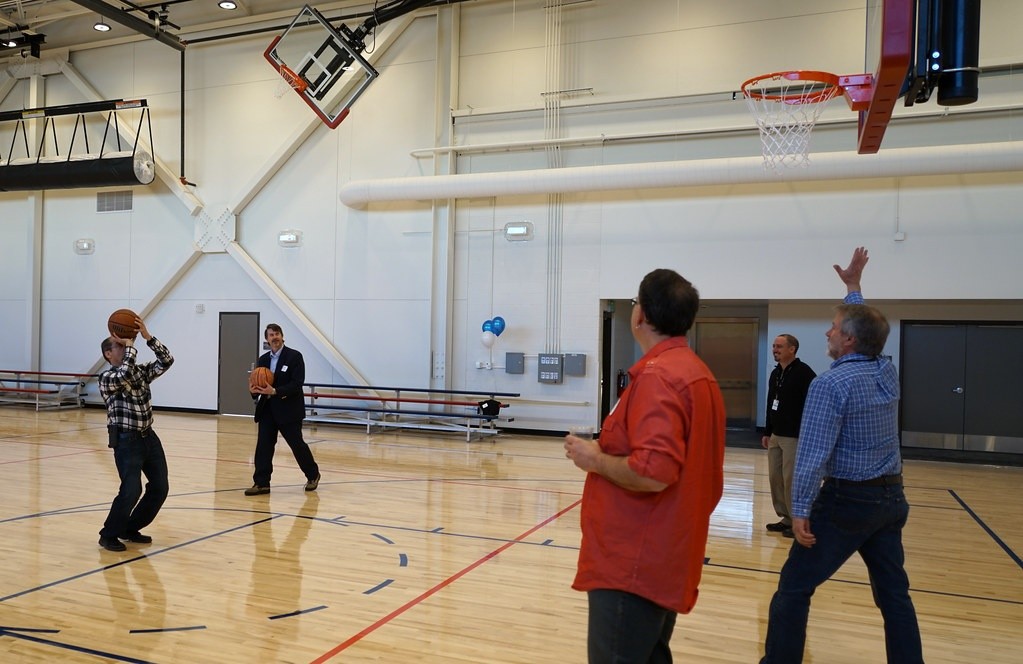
98;535;127;551
245;484;270;495
305;472;321;491
119;531;152;542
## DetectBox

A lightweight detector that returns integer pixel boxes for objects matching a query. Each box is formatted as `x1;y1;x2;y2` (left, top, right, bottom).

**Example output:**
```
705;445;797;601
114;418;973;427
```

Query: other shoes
782;524;794;538
766;522;783;532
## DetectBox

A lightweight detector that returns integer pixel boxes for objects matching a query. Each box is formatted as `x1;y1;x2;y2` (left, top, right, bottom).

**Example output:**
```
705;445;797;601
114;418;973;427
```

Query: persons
761;334;817;538
245;324;321;495
755;244;926;664
98;317;174;551
565;268;726;664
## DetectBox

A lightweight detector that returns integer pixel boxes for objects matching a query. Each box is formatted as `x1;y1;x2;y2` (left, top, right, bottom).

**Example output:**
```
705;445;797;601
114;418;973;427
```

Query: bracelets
272;388;275;394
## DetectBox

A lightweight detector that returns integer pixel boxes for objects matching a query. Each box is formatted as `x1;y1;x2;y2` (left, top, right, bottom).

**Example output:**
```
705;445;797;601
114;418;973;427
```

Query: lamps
218;0;237;10
279;234;297;243
2;26;17;47
93;15;112;32
78;242;92;250
507;226;527;236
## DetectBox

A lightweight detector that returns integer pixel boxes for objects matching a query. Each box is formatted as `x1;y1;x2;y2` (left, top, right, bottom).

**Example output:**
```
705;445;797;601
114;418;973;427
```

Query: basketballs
250;367;274;390
107;308;141;340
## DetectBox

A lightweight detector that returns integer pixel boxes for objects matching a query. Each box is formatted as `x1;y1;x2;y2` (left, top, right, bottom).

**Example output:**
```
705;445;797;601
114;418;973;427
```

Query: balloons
482;320;492;332
491;317;505;337
480;331;494;349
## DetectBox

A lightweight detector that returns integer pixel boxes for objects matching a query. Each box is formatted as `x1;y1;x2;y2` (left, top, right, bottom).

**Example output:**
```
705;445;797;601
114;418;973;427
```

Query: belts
119;426;151;438
824;473;903;487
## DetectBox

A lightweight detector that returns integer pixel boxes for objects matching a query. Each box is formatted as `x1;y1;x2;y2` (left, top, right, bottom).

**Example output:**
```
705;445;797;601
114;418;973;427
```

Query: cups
567;424;594;442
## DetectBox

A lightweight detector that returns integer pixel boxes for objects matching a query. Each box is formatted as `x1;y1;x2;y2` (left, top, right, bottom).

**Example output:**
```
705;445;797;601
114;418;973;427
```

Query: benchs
302;383;521;444
0;370;99;411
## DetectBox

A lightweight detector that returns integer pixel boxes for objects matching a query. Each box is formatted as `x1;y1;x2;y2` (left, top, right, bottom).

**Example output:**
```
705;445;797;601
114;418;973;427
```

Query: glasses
630;298;648;320
